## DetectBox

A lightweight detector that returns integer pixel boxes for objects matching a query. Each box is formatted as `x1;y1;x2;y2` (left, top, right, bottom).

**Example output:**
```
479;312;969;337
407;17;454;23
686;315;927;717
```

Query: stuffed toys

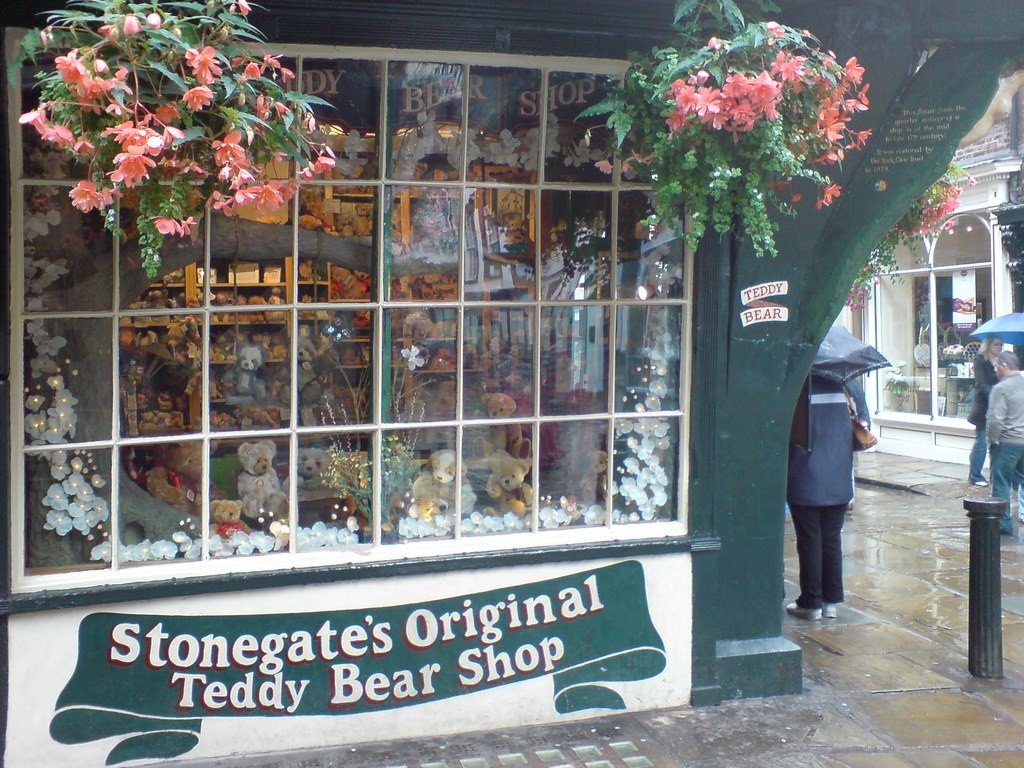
121;258;612;538
295;154;529;255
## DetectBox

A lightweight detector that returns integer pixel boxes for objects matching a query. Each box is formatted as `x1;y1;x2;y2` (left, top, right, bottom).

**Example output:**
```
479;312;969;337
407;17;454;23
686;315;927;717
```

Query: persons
786;376;871;622
986;351;1024;536
968;336;1003;487
952;297;976;315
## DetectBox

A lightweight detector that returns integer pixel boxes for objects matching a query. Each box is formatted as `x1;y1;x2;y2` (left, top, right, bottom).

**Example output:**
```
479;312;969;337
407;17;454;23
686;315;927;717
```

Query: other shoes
787;602;823;621
972;481;988;487
821;601;838;618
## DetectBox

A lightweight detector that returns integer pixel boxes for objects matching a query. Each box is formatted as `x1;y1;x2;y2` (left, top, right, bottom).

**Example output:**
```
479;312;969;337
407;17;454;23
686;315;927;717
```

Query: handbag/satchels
844;386;878;451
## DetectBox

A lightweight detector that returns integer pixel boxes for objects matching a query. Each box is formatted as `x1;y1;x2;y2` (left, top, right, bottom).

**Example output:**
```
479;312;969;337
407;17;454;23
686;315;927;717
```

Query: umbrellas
806;326;893;389
969;312;1024;346
